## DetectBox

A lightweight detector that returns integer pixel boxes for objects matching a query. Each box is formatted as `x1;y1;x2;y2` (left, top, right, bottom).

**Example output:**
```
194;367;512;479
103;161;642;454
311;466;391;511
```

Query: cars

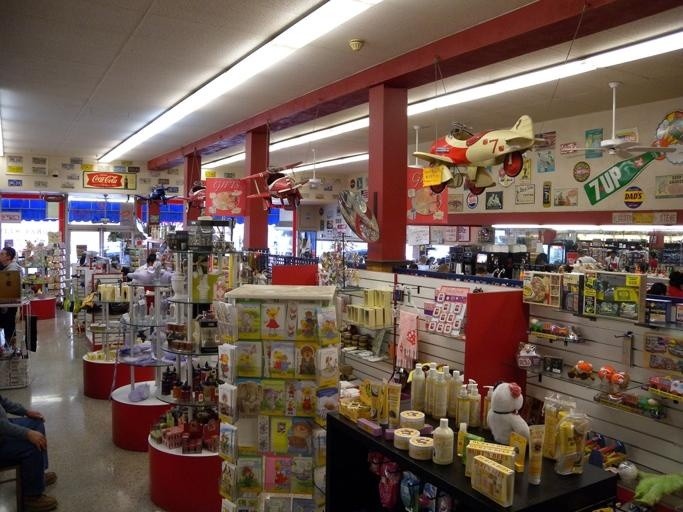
339;249;369;270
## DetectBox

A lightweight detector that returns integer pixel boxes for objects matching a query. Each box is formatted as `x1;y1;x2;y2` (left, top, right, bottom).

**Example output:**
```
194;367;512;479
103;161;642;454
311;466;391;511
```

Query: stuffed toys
487;381;530;445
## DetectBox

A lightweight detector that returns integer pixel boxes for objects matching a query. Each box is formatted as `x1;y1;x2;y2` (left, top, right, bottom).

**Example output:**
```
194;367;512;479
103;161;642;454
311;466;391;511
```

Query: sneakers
17;471;58;512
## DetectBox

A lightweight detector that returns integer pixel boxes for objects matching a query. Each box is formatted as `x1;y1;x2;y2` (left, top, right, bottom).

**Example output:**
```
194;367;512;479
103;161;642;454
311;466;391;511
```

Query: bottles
411;362;467;466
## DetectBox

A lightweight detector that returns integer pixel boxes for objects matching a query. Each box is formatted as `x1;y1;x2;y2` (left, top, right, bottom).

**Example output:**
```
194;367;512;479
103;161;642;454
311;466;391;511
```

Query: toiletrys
376;378;387;430
388;383;401;429
528;424;545;483
411;360;494;428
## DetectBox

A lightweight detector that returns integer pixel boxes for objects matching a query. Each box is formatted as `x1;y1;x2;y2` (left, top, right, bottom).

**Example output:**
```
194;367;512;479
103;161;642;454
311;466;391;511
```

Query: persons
121;251;131;282
0;393;58;511
399;255;449;279
474;248;683;297
141;254;157;269
0;247;23;349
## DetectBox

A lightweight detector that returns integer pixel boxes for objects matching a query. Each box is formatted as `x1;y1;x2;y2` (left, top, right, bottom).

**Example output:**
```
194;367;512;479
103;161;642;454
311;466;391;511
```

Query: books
217;301;340;512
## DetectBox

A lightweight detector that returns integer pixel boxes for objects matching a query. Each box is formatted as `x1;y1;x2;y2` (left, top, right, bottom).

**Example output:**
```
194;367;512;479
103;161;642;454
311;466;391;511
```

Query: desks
326;399;622;510
